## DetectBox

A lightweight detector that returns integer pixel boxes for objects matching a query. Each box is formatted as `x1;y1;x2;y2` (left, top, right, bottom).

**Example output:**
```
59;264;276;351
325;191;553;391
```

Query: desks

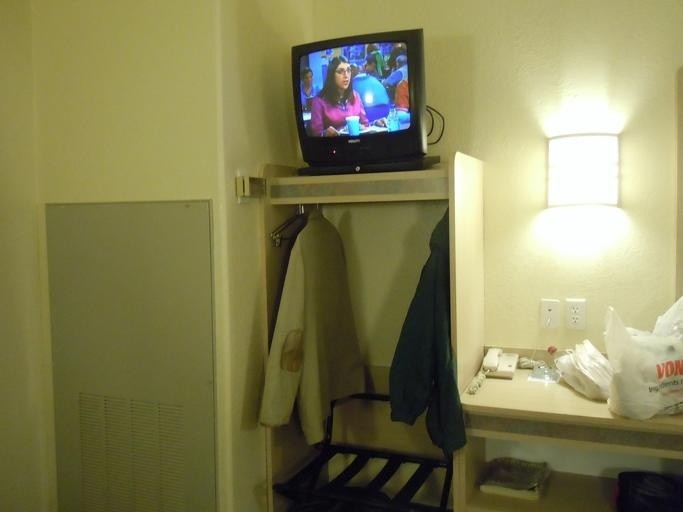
456;346;683;511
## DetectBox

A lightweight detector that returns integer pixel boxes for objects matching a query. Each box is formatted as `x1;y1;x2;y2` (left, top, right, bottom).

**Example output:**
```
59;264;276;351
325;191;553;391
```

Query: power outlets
561;296;588;331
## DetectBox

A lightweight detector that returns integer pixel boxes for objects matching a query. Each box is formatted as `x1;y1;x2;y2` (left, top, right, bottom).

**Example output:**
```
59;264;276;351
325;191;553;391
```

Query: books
475;457;551;501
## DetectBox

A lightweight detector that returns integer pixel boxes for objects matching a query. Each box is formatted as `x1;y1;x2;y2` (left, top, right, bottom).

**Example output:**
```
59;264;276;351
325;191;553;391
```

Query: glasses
334;67;352;75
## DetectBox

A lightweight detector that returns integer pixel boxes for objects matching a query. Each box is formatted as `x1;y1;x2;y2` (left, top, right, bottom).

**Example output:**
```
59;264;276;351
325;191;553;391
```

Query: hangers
269;204;308;242
304;204;325;220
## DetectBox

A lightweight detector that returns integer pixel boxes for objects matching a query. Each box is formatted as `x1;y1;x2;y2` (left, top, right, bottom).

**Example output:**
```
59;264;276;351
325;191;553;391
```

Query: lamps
545;131;622;209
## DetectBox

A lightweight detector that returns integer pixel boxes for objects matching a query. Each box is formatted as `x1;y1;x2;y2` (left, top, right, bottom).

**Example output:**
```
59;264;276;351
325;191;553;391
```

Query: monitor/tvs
291;28;440;175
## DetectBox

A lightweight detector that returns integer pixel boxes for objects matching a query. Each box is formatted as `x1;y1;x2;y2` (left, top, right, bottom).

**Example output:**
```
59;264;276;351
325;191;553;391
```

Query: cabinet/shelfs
254;150;485;512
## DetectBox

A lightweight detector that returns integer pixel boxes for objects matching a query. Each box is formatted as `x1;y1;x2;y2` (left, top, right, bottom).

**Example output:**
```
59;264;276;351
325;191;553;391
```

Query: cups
345;116;359;137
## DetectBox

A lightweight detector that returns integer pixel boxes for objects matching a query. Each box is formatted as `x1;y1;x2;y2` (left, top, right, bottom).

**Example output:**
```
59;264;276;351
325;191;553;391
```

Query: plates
339;125;388;134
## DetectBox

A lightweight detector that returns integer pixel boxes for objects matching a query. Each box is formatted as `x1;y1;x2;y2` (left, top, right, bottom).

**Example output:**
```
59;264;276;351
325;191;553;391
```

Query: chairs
273;392;454;512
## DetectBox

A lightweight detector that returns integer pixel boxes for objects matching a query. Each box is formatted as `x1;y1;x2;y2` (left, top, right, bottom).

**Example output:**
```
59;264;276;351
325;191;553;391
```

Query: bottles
387;104;400;132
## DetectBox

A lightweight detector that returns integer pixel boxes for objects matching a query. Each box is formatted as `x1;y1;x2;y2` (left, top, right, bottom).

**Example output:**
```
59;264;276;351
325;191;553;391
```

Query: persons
299;42;410;137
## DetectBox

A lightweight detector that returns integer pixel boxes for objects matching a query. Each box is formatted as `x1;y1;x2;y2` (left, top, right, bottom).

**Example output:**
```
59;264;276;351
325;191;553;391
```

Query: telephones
467;346;519;380
